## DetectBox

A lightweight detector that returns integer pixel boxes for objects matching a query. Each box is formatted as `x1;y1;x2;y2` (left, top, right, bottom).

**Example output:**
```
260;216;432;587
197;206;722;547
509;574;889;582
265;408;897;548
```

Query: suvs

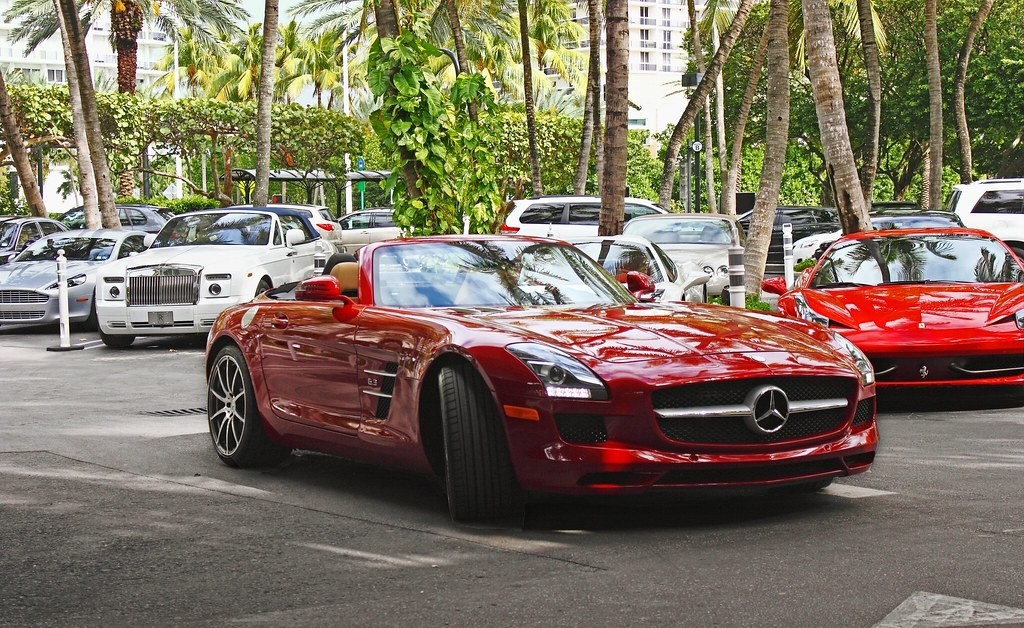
944;179;1024;268
500;194;674;248
48;203;183;244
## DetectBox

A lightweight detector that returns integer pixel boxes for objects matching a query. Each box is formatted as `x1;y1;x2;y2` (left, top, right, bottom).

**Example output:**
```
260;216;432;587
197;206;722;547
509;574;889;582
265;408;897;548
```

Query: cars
550;202;975;308
0;229;155;331
0;216;70;269
332;209;455;256
225;202;347;255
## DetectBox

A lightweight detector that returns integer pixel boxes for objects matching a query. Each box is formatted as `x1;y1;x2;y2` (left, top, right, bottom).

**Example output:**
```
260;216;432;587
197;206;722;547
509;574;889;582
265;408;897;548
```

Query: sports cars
205;230;883;523
760;225;1024;391
91;207;341;350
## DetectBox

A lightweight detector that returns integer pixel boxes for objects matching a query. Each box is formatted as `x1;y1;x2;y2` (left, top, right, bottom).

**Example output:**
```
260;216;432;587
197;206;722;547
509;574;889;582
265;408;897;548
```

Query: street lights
681;72;710;213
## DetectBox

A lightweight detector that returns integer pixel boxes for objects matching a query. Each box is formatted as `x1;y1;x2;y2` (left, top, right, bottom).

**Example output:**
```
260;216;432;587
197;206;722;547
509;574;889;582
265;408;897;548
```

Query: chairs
330;262;358;304
617;251;647;275
458;262;507;304
322;253;358;297
701;226;725;241
